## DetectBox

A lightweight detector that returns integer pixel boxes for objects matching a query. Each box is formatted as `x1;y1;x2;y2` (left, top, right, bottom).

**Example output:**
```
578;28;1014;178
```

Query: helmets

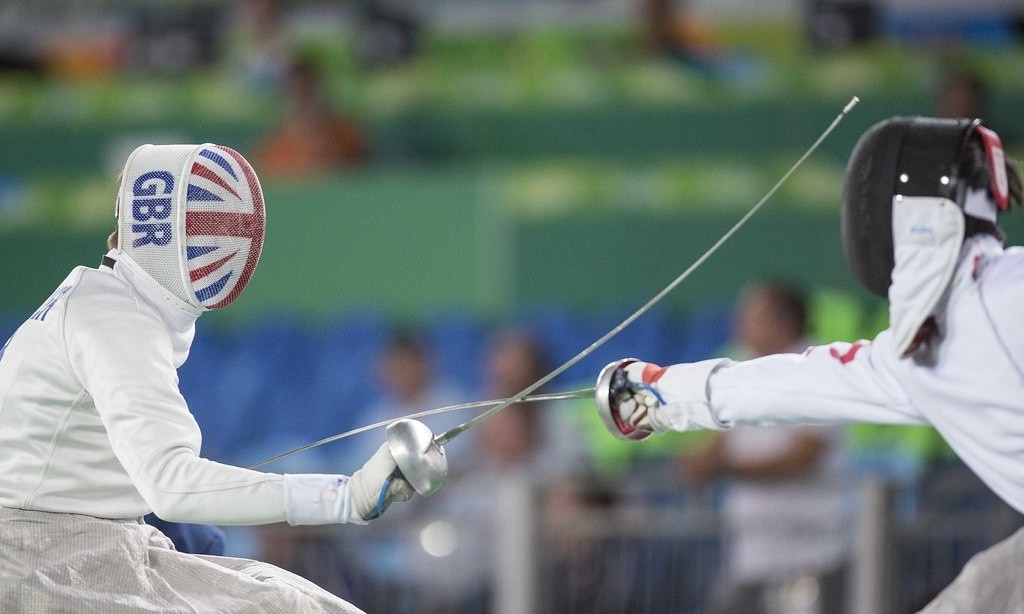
841;113;1009;300
118;142;266;310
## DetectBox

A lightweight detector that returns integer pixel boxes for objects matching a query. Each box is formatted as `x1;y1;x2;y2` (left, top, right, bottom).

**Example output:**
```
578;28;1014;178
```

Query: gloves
282;441;416;528
613;357;734;433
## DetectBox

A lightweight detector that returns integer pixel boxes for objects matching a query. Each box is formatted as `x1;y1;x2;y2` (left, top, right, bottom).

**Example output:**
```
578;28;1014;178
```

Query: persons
0;2;421;181
344;274;861;614
606;111;1023;614
0;143;414;614
